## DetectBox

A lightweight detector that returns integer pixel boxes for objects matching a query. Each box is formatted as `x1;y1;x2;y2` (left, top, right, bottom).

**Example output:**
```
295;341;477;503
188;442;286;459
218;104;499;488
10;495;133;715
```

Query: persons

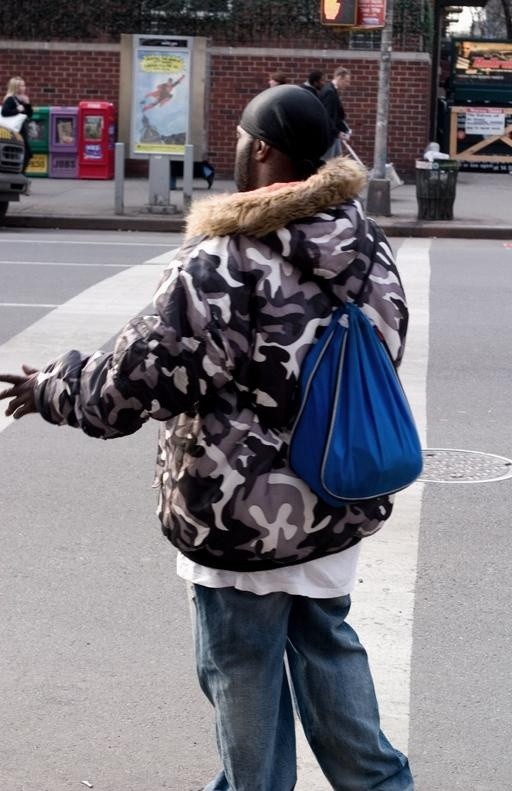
0;80;416;791
267;66;354;160
1;76;39;196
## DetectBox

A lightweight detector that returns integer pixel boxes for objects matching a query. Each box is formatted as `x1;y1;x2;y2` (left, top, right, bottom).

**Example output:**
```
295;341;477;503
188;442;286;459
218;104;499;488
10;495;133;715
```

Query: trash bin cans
416;159;458;221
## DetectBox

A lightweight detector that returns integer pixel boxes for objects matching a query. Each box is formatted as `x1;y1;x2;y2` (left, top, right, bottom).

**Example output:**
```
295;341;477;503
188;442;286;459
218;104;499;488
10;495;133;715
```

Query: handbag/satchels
0;105;28;133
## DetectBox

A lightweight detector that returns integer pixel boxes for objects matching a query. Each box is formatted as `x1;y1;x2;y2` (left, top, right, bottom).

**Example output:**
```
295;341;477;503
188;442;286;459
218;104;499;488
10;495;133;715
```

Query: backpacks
261;221;423;506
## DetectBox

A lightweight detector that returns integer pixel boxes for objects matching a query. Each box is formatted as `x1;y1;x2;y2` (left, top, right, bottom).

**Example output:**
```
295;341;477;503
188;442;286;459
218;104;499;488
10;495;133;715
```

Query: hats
239;83;338;162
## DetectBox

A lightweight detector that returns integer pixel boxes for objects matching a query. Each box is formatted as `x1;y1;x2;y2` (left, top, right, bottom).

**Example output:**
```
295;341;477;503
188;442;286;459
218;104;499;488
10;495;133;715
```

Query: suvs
1;124;28;224
